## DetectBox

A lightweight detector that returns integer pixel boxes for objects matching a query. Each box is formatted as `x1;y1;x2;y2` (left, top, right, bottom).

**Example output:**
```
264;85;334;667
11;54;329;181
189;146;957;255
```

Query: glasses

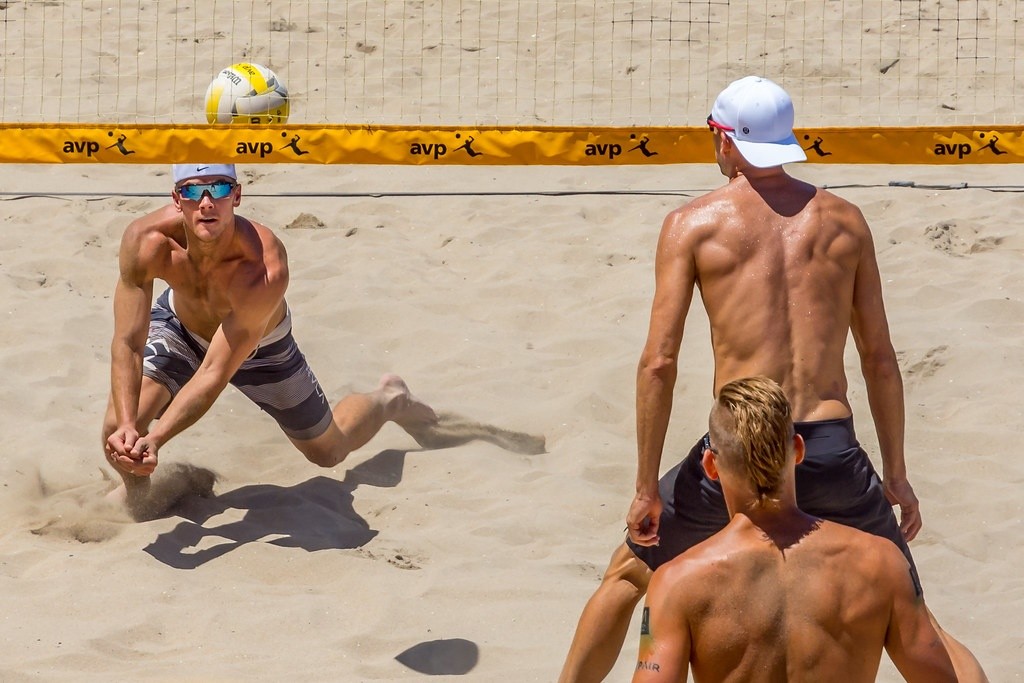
702;432;718;455
175;180;237;202
707;113;735;132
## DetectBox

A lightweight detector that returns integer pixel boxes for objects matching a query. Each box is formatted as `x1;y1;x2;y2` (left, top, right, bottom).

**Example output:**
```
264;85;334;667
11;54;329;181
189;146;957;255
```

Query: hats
711;76;807;168
172;164;237;183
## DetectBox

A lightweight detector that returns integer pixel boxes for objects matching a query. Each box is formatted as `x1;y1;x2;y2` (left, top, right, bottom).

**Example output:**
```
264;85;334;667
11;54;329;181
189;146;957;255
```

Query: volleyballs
204;61;290;124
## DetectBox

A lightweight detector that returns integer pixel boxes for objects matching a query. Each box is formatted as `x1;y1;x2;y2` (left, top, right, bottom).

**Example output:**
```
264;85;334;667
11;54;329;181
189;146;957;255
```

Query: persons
554;76;989;683
102;163;437;487
634;377;956;683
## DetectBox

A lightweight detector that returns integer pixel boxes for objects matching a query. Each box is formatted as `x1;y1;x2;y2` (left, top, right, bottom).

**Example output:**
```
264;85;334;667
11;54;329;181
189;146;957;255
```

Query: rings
131;470;134;473
